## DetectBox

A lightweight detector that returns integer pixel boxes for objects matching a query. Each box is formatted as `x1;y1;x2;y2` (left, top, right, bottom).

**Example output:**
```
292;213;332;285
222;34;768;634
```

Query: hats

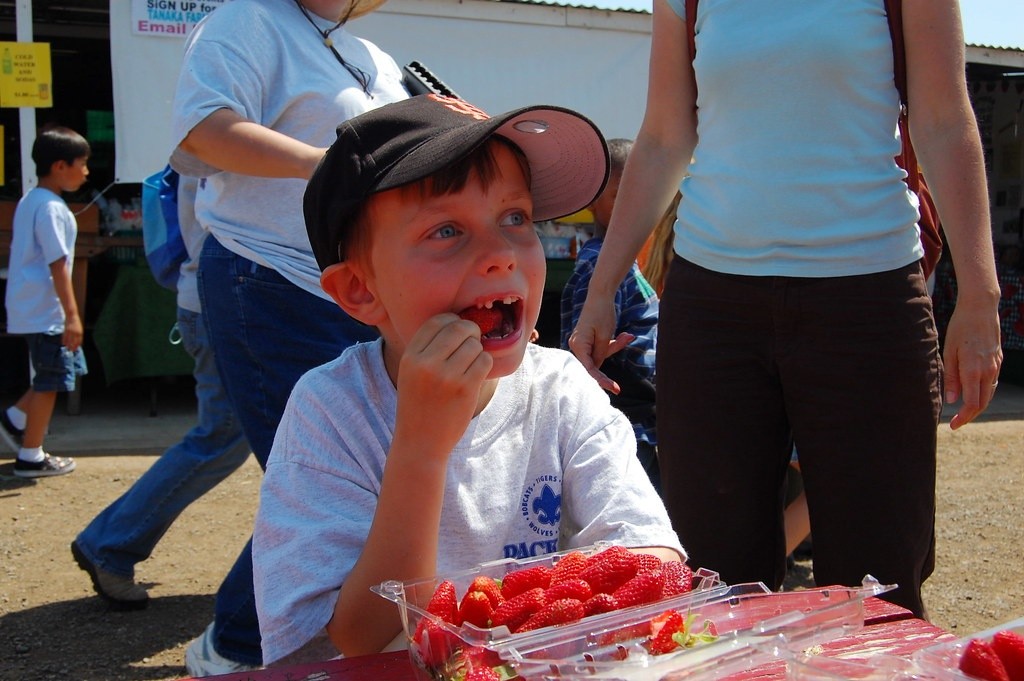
303;94;610;273
340;0;388;21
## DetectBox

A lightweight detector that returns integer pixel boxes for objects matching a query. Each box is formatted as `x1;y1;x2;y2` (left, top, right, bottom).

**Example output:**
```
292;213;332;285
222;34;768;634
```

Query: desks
0;229;148;419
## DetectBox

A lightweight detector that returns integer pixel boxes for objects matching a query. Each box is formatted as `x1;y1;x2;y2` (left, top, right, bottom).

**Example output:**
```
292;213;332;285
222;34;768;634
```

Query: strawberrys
459;304;502;334
410;545;694;681
960;629;1024;681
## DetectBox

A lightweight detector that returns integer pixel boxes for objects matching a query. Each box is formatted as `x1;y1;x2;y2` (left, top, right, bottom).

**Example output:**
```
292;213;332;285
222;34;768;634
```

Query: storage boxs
178;540;1024;681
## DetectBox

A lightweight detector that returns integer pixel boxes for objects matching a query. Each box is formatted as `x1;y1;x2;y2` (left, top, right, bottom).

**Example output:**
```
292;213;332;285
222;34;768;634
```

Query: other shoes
0;408;23;454
13;451;76;477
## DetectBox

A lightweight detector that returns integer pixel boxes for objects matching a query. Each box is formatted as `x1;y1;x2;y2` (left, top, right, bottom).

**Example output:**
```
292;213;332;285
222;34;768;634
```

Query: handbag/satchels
145;163;193;295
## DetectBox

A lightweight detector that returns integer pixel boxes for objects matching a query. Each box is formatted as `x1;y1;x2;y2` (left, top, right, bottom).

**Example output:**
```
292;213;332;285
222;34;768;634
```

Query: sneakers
185;620;256;678
71;540;150;606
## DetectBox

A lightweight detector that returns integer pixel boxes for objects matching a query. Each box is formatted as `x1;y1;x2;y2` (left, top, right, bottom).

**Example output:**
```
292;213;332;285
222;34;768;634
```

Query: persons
558;0;1004;624
251;95;689;659
0;126;90;478
70;0;415;678
